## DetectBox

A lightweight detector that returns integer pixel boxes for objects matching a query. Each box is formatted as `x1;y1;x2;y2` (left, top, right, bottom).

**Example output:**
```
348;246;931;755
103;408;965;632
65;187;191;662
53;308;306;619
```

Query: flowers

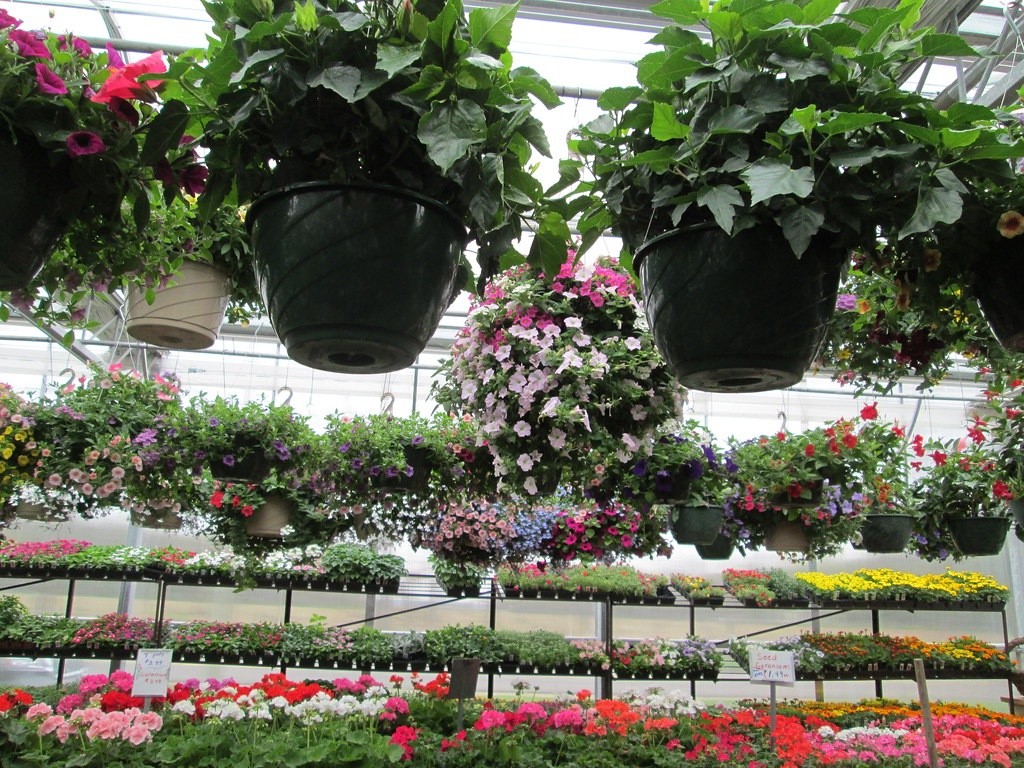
1;597;1013;673
1;0;1024;597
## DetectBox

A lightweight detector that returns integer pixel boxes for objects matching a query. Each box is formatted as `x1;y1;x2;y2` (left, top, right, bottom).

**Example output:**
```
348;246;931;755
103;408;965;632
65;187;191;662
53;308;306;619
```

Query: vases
125;254;231;351
0;643;1016;678
763;504;815;551
668;504;724;545
859;512;914;554
636;224;846;390
949;515;1010;554
0;564;1005;612
250;177;471;373
973;234;1024;352
695;534;735;559
